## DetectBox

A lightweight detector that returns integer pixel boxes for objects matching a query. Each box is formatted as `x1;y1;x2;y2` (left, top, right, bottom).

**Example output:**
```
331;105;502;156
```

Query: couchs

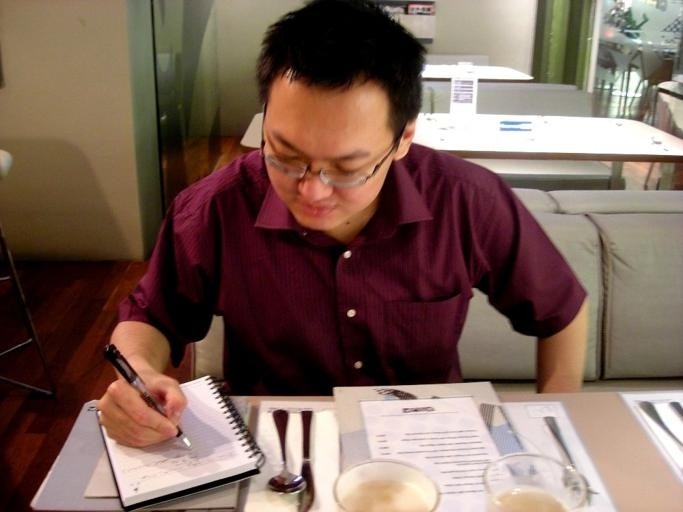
416;78;612;188
187;188;682;393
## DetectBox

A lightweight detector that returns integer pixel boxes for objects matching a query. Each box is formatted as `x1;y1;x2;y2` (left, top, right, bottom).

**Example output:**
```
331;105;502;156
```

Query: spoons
267;409;308;496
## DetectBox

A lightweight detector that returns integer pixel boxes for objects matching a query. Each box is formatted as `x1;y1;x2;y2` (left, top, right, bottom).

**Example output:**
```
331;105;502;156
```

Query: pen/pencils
102;345;195;447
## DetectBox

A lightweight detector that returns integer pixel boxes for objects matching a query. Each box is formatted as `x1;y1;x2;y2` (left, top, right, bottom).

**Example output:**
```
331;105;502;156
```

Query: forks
544;414;598;499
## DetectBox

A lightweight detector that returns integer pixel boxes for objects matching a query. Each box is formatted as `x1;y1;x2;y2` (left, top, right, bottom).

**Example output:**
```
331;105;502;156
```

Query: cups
480;453;586;512
331;461;442;512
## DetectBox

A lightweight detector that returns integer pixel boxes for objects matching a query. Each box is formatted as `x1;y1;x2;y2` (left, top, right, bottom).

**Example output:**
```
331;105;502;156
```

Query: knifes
297;411;315;512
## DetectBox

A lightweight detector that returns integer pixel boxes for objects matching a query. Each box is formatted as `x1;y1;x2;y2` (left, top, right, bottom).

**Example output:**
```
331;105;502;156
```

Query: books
94;373;267;512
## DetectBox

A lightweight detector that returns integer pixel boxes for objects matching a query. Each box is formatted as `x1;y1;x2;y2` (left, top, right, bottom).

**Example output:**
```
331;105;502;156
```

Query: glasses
260;101;405;187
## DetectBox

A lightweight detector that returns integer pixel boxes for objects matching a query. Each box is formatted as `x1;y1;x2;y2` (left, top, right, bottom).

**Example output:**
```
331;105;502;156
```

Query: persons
94;0;590;449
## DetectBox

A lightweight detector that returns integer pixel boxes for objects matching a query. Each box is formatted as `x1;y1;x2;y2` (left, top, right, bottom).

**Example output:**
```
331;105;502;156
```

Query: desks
416;60;535;82
238;108;683;192
30;390;683;512
